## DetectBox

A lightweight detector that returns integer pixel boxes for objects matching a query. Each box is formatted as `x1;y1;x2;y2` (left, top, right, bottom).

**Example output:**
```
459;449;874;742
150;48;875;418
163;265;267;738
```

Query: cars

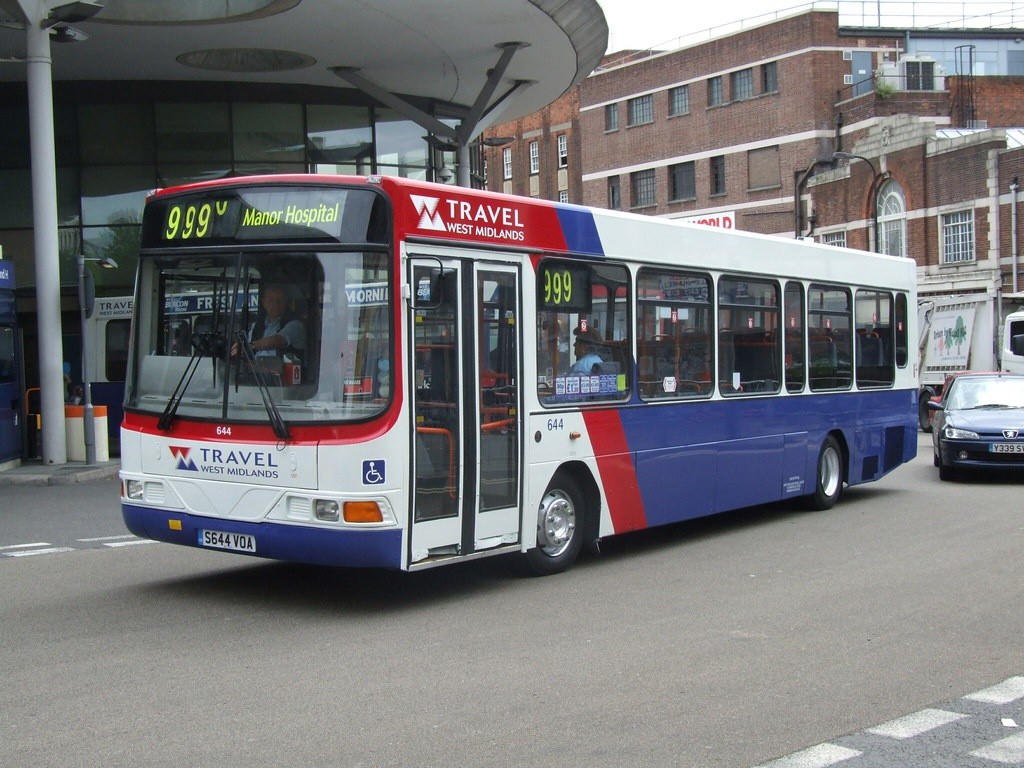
927;368;1017;434
926;375;1024;479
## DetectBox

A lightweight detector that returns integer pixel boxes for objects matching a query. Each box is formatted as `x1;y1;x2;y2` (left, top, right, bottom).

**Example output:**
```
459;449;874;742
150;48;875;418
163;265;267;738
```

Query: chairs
595;322;905;398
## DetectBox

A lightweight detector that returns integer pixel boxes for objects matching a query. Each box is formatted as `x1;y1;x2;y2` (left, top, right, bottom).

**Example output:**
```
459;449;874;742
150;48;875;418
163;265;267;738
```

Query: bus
115;173;922;575
0;246;25;466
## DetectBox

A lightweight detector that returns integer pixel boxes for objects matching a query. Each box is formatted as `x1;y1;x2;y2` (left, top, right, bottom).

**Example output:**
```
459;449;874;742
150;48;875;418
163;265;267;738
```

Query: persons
232;279;306;361
567;326;601;377
30;360;72;461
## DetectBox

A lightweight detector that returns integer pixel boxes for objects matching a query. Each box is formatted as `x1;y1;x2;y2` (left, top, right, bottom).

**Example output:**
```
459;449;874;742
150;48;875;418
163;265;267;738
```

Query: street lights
830;152;882;254
794;157;838;239
77;255;118;466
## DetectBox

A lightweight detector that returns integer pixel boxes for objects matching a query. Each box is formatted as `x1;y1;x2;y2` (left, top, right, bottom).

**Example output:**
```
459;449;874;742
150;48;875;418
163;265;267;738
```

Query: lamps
422;135;461;152
43;1;104;45
470;134;518;148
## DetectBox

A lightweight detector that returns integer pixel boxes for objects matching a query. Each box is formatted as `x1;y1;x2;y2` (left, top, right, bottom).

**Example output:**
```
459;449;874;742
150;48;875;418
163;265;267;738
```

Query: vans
998;311;1024;376
